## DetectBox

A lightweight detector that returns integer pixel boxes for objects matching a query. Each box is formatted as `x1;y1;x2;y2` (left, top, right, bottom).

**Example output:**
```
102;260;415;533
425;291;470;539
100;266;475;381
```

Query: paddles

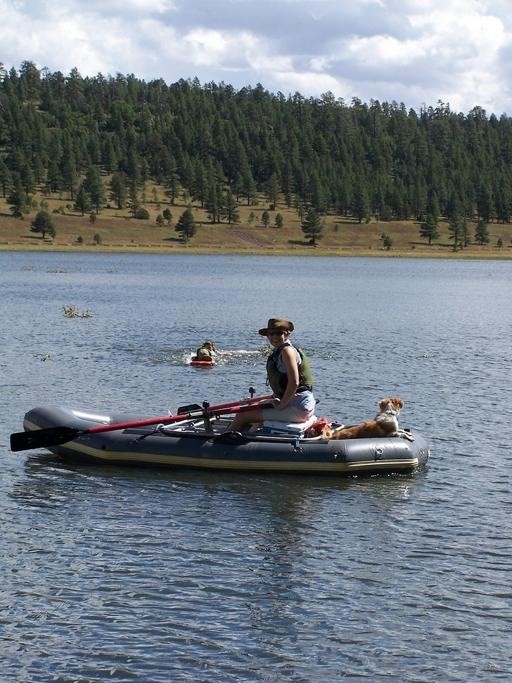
11;398;276;453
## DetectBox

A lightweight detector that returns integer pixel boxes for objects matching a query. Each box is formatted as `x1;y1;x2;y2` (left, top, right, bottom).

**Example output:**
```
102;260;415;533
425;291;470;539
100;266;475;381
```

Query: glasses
269;330;283;336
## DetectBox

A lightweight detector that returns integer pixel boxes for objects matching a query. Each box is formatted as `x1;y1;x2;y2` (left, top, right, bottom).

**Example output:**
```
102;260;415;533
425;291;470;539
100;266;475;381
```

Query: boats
190;353;217;369
20;403;432;480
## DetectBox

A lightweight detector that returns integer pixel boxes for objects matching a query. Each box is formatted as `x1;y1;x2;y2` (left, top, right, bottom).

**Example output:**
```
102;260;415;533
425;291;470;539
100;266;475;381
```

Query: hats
259;318;294;335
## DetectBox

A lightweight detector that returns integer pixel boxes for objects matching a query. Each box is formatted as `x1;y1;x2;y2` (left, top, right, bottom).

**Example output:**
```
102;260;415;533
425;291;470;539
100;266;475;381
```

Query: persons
226;318;317;433
203;341;217;356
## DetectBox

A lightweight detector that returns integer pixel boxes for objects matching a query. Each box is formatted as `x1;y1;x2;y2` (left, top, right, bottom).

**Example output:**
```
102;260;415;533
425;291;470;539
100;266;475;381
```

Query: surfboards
190;360;215;366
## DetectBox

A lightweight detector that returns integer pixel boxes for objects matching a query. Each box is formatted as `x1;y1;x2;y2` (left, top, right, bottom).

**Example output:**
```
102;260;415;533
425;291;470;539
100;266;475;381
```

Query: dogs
322;397;415;442
